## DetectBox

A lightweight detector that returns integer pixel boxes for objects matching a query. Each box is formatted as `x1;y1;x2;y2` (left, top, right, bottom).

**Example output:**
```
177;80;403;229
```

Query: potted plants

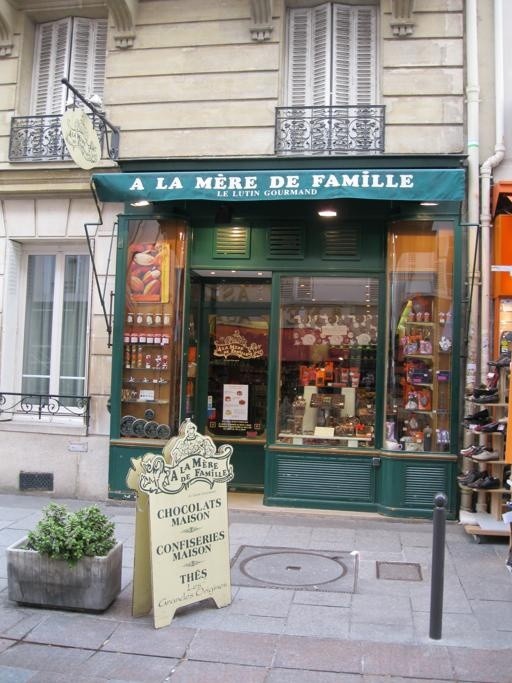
4;501;123;613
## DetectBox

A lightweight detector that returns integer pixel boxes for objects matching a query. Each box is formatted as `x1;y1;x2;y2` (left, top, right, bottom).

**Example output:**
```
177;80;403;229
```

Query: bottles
124;311;173;368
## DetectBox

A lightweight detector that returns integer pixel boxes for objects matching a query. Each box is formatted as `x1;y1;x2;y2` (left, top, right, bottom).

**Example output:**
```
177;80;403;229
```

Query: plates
119;408;171;439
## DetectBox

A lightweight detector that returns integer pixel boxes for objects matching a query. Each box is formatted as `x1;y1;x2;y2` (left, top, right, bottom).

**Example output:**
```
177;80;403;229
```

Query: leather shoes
456;351;512;489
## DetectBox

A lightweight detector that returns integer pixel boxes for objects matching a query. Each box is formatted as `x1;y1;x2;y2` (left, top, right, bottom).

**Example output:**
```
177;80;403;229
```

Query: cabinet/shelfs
458;363;511;543
402;293;440;452
121;320;172;435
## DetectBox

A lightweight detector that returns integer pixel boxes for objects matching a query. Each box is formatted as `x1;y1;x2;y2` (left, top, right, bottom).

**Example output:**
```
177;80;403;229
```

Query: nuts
130;250;161;295
